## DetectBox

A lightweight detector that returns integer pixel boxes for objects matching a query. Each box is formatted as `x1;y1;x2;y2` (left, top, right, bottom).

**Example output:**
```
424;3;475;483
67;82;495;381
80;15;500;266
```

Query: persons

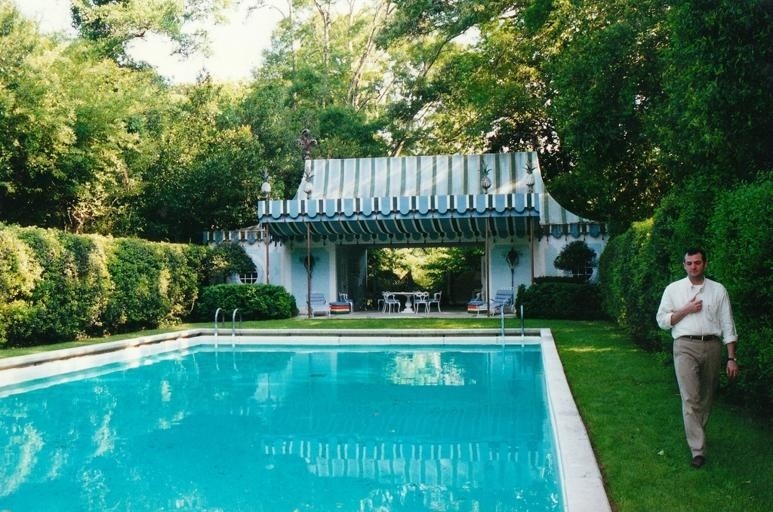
654;247;739;469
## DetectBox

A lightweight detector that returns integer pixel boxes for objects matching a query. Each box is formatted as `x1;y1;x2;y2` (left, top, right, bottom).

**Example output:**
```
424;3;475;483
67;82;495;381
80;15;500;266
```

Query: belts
680;333;718;341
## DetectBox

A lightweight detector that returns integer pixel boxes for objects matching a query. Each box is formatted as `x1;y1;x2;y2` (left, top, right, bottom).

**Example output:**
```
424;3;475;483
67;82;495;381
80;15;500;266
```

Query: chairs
466;288;516;316
382;290;442;315
306;290;354;319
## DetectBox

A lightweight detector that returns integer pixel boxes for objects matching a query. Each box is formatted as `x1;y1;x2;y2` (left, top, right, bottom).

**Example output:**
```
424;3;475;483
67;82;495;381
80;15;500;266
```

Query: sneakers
690;454;704;467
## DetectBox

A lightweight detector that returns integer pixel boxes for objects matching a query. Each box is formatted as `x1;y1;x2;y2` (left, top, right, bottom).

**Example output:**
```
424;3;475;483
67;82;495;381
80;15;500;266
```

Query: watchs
726;357;736;362
